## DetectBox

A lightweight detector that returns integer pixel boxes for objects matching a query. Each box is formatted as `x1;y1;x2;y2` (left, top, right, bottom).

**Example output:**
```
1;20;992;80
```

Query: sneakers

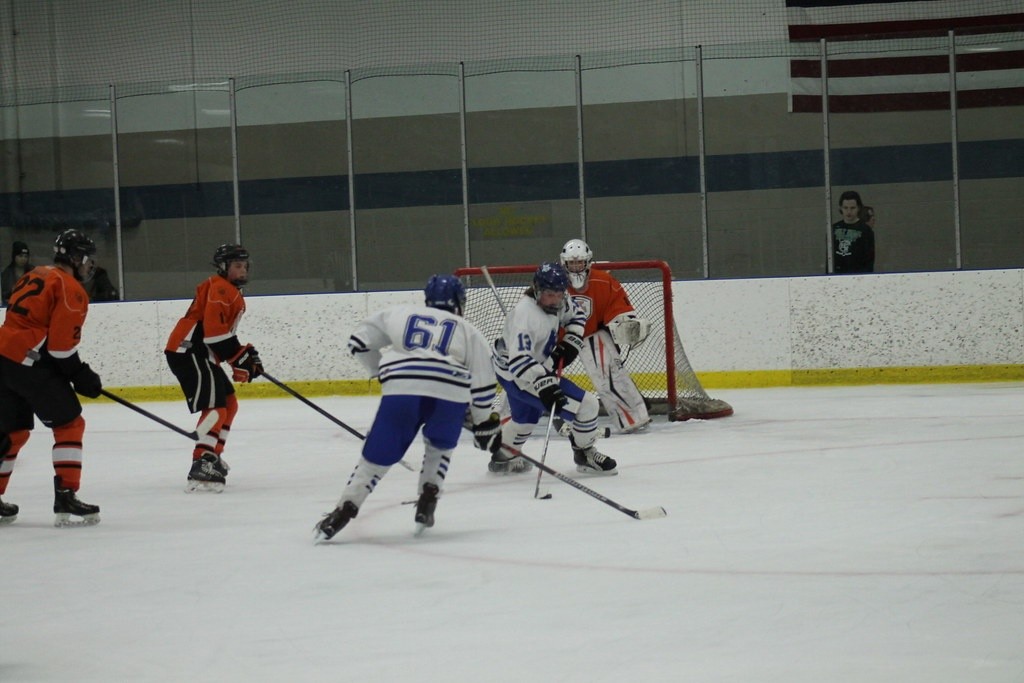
184;451;226;492
571;446;618;476
53;475;101;526
488;456;534;476
0;498;19;526
313;501;358;546
212;453;230;476
414;482;439;536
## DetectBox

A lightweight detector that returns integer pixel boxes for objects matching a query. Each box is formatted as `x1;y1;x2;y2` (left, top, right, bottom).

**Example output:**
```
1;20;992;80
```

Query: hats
11;241;29;256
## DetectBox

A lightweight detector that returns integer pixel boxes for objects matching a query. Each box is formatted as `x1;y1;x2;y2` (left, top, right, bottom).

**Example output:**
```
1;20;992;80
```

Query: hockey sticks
481;265;611;439
262;371;414;472
463;421;667;518
102;390;197;442
532;333;567;500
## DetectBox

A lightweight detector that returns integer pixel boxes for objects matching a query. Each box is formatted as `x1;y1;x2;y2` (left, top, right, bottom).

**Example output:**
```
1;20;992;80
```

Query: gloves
533;373;568;413
74;361;102;399
551;333;587;369
230;343;264;384
472;413;502;452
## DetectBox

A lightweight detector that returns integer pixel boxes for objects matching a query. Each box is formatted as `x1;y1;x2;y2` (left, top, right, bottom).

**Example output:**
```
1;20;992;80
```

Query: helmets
534;261;567;314
424;274;466;313
54;228;98;265
214;242;250;284
560;239;593;288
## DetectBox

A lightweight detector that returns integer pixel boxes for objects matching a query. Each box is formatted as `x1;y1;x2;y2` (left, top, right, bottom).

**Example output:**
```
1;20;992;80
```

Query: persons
0;242;36;307
0;230;102;516
165;242;264;484
85;268;118;302
314;274;502;539
561;239;652;436
825;191;874;275
493;262;617;473
